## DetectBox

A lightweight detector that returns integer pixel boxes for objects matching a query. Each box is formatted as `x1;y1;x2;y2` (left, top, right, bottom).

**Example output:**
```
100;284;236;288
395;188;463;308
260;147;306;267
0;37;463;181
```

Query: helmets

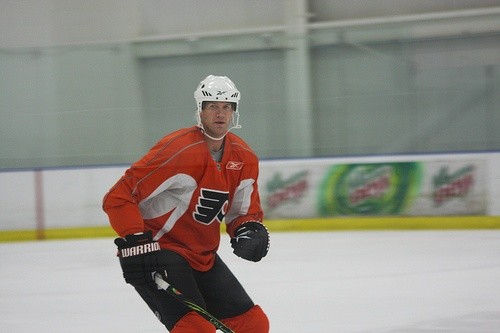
194;75;241;112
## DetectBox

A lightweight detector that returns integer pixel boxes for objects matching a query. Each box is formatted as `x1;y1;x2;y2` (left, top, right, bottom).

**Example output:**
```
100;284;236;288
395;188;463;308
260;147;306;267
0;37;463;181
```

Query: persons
101;74;270;333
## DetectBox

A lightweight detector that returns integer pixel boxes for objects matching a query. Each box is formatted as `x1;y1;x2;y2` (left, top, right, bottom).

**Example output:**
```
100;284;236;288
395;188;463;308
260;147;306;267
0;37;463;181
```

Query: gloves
114;230;165;286
230;222;269;262
